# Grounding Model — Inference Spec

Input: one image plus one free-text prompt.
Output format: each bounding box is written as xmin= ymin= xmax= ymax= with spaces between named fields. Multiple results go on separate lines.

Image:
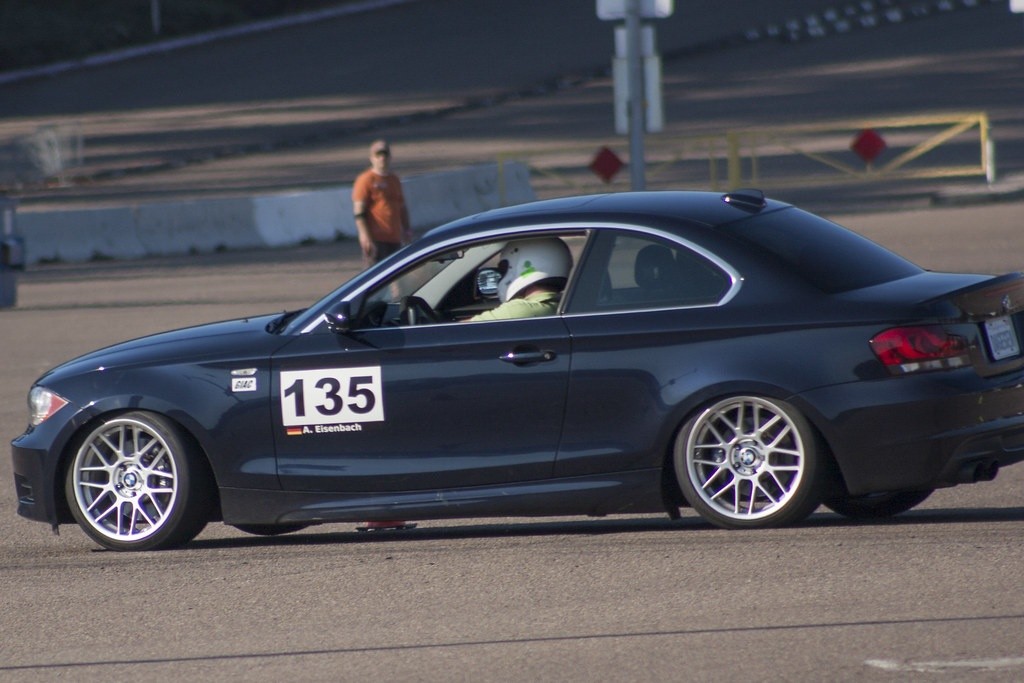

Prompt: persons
xmin=352 ymin=140 xmax=413 ymax=266
xmin=459 ymin=237 xmax=574 ymax=322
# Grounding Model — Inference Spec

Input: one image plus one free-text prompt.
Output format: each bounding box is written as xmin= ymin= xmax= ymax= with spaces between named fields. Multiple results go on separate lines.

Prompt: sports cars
xmin=9 ymin=186 xmax=1023 ymax=555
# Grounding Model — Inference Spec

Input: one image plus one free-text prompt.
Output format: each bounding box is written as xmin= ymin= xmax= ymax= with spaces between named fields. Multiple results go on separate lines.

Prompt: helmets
xmin=497 ymin=236 xmax=573 ymax=304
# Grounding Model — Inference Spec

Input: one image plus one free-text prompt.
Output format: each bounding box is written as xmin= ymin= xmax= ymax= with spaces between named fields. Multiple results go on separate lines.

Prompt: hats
xmin=371 ymin=140 xmax=390 ymax=155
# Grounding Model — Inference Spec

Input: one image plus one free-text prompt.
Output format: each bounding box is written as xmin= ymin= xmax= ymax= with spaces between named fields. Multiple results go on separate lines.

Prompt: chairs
xmin=611 ymin=245 xmax=680 ymax=307
